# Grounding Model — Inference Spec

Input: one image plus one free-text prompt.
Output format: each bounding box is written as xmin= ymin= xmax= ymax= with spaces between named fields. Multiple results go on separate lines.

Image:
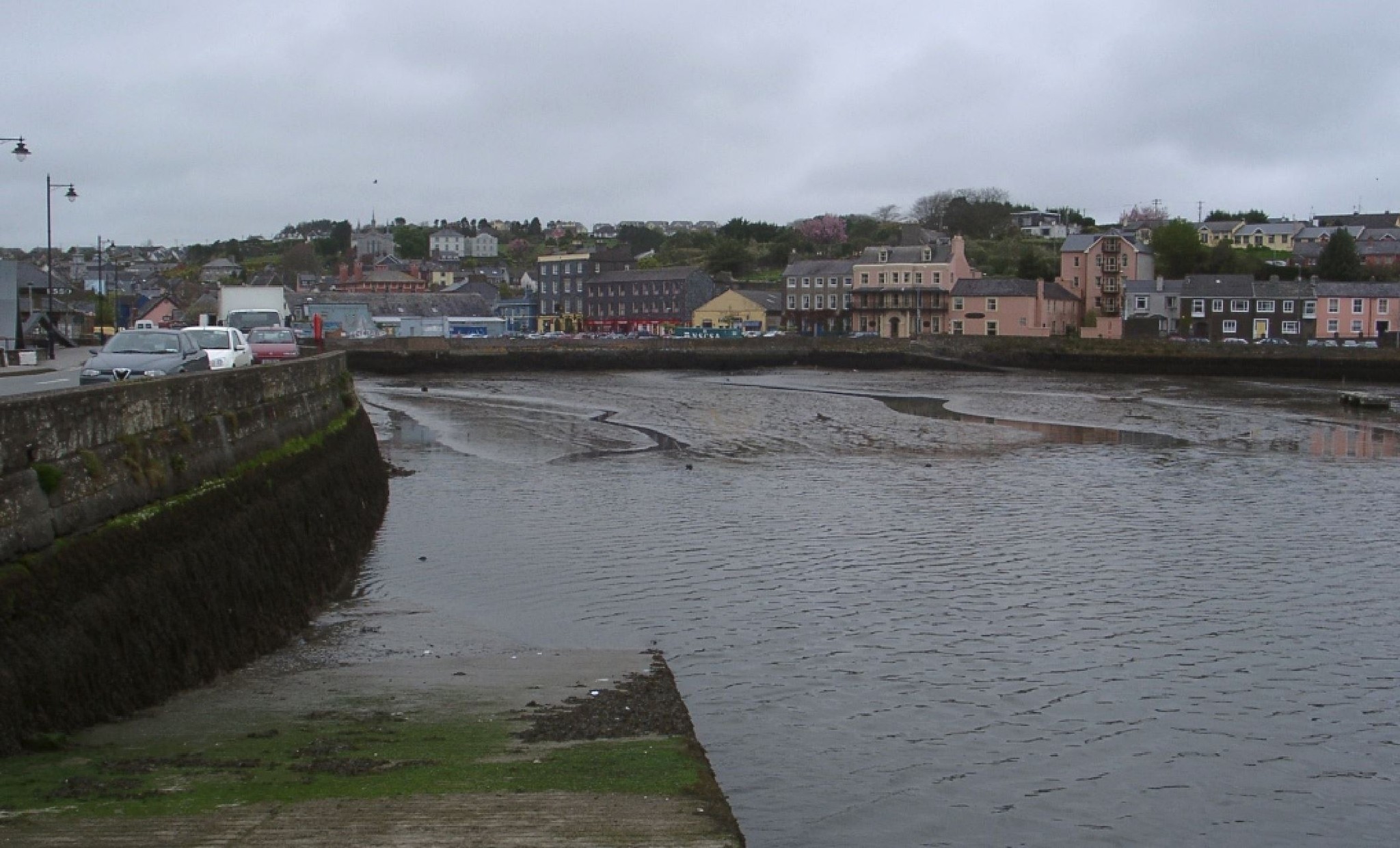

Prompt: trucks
xmin=215 ymin=284 xmax=288 ymax=345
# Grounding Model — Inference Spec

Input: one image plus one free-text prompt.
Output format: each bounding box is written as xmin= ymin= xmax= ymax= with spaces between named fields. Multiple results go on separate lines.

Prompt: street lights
xmin=97 ymin=233 xmax=117 ymax=351
xmin=46 ymin=173 xmax=80 ymax=359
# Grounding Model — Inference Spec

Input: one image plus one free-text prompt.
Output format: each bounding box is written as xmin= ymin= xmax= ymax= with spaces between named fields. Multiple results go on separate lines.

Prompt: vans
xmin=134 ymin=319 xmax=153 ymax=330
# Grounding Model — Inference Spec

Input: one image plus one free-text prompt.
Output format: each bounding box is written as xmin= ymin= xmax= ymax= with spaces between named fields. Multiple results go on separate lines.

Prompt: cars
xmin=839 ymin=331 xmax=878 ymax=340
xmin=520 ymin=330 xmax=663 ymax=340
xmin=1306 ymin=340 xmax=1318 ymax=346
xmin=1362 ymin=340 xmax=1379 ymax=349
xmin=242 ymin=326 xmax=300 ymax=367
xmin=176 ymin=325 xmax=254 ymax=373
xmin=78 ymin=328 xmax=211 ymax=386
xmin=1341 ymin=340 xmax=1359 ymax=348
xmin=1224 ymin=336 xmax=1291 ymax=345
xmin=461 ymin=333 xmax=486 ymax=339
xmin=743 ymin=329 xmax=786 ymax=338
xmin=1325 ymin=340 xmax=1340 ymax=347
